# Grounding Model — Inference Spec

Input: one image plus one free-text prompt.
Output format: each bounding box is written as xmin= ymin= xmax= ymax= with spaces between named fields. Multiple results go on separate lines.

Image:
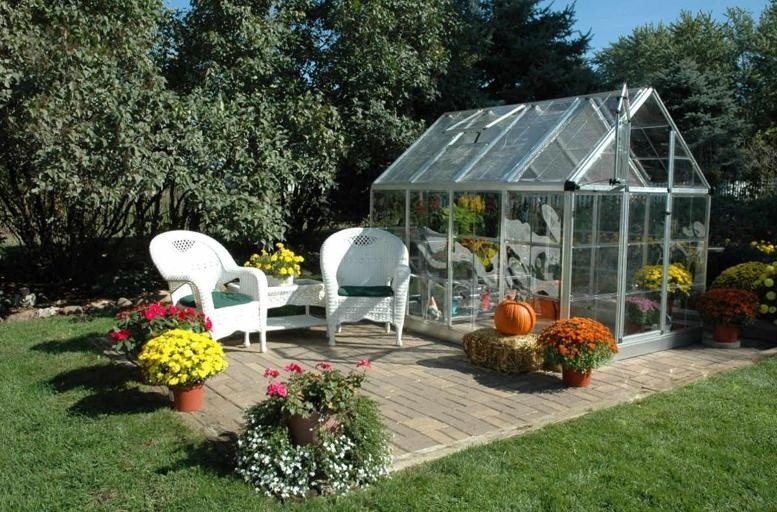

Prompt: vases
xmin=287 ymin=410 xmax=350 ymax=456
xmin=171 ymin=383 xmax=205 ymax=413
xmin=266 ymin=274 xmax=294 ymax=287
xmin=713 ymin=318 xmax=740 ymax=343
xmin=560 ymin=360 xmax=592 ymax=388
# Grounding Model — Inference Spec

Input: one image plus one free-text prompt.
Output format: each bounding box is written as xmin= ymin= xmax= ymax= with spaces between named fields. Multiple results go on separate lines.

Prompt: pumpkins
xmin=494 ymin=300 xmax=536 ymax=336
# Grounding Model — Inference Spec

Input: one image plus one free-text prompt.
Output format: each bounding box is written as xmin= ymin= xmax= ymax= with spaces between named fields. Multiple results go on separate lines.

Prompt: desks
xmin=228 ymin=279 xmax=328 ymax=349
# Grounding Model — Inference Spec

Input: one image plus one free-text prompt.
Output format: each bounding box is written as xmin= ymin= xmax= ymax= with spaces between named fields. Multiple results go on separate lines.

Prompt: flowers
xmin=536 ymin=316 xmax=620 ymax=376
xmin=697 ymin=288 xmax=761 ymax=326
xmin=625 ymin=261 xmax=694 ymax=327
xmin=259 ymin=354 xmax=371 ymax=417
xmin=107 ymin=301 xmax=231 ymax=391
xmin=243 ymin=240 xmax=305 ymax=279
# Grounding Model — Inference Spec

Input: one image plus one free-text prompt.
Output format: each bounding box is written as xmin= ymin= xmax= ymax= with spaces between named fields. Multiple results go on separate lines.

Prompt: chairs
xmin=147 ymin=228 xmax=269 ymax=354
xmin=319 ymin=225 xmax=413 ymax=348
